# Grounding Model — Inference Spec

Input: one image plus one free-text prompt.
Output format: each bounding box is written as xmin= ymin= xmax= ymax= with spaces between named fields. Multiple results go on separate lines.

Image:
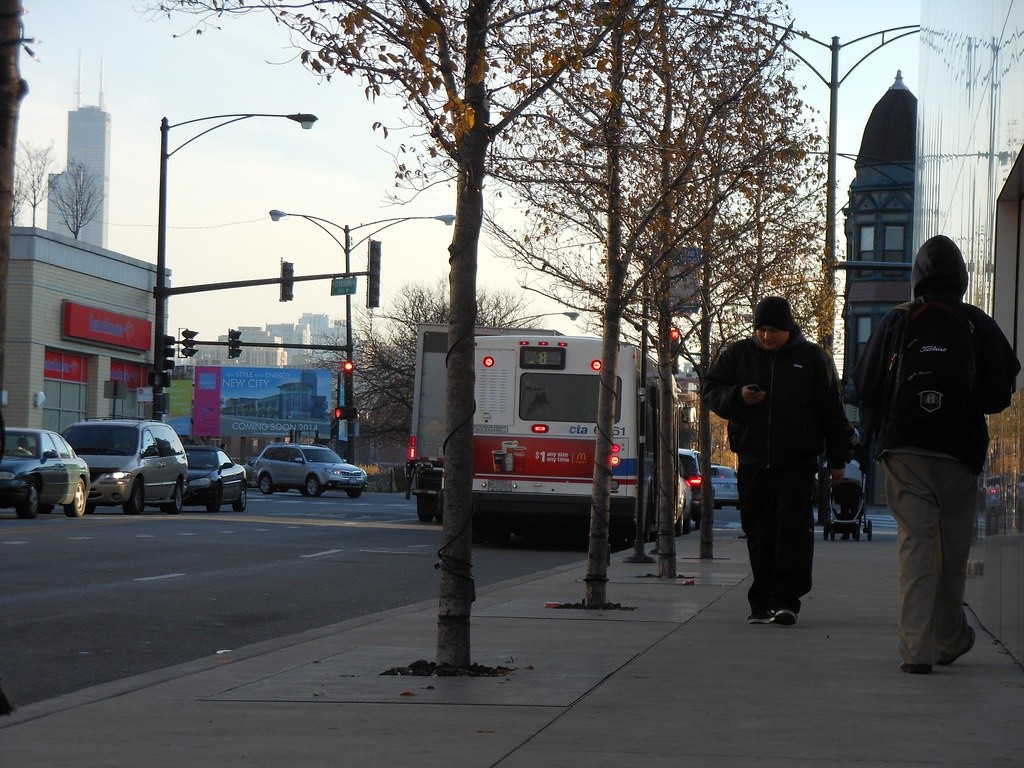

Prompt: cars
xmin=240 ymin=456 xmax=289 ymax=493
xmin=0 ymin=427 xmax=91 ymax=520
xmin=182 ymin=444 xmax=248 ymax=513
xmin=675 ymin=445 xmax=741 ymax=538
xmin=984 ymin=474 xmax=1024 ymax=508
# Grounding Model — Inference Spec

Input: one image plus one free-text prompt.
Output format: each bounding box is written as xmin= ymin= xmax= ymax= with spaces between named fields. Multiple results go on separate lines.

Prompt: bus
xmin=472 ymin=334 xmax=682 ymax=547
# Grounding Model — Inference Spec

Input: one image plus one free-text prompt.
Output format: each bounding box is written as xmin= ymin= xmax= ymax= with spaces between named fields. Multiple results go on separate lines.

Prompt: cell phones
xmin=748 ymin=384 xmax=760 ymax=393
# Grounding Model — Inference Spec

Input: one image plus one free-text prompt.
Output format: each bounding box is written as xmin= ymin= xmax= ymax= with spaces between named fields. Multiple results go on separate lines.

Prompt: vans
xmin=56 ymin=415 xmax=188 ymax=515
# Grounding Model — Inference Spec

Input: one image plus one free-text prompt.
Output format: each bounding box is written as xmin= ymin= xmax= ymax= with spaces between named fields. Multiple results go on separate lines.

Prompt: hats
xmin=754 ymin=297 xmax=792 ymax=329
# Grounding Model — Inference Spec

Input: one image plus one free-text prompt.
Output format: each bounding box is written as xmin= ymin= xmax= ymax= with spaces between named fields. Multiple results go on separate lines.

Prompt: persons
xmin=841 ymin=428 xmax=864 ymax=541
xmin=702 ymin=296 xmax=850 ymax=624
xmin=851 ymin=234 xmax=1022 ymax=675
xmin=18 ymin=437 xmax=35 ymax=454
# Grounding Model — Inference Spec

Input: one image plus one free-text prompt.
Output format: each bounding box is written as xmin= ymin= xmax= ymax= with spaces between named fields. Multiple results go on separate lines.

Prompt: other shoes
xmin=776 ymin=608 xmax=797 ymax=625
xmin=841 ymin=532 xmax=850 ymax=540
xmin=934 ymin=625 xmax=974 ymax=666
xmin=748 ymin=606 xmax=775 ymax=624
xmin=899 ymin=661 xmax=931 ymax=673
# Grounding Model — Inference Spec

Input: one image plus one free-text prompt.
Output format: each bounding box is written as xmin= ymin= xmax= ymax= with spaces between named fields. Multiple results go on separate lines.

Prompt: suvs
xmin=254 ymin=442 xmax=368 ymax=499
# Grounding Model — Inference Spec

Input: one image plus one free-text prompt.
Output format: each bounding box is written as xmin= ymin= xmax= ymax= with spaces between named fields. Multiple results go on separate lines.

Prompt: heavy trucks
xmin=406 ymin=323 xmax=566 ymax=523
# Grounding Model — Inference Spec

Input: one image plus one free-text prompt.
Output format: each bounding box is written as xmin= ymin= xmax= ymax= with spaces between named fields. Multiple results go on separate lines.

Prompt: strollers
xmin=823 ymin=440 xmax=874 ymax=542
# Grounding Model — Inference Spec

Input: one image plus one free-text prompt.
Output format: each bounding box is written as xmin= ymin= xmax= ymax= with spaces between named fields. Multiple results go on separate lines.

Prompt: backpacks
xmin=876 ymin=301 xmax=980 ymax=445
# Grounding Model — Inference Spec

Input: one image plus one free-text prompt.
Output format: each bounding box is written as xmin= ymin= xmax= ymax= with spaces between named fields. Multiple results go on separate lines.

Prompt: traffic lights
xmin=345 ymin=362 xmax=353 ymax=406
xmin=229 ymin=329 xmax=243 ymax=358
xmin=152 ymin=371 xmax=171 ymax=387
xmin=160 ymin=334 xmax=176 ymax=369
xmin=335 ymin=407 xmax=347 ymax=419
xmin=181 ymin=329 xmax=200 ymax=357
xmin=670 ymin=330 xmax=679 ymax=372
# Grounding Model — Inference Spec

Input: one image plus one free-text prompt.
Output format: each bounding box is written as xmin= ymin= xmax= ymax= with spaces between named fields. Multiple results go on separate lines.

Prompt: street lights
xmin=269 ymin=209 xmax=458 ymax=463
xmin=152 ymin=110 xmax=317 ymax=421
